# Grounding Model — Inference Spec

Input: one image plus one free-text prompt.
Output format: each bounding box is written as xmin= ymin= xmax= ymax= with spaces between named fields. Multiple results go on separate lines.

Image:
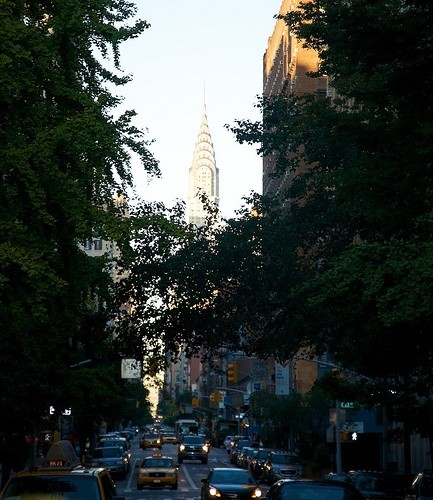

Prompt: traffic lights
xmin=340 ymin=430 xmax=347 ymax=442
xmin=227 ymin=362 xmax=238 ymax=386
xmin=348 ymin=431 xmax=359 ymax=443
xmin=210 ymin=391 xmax=224 ymax=405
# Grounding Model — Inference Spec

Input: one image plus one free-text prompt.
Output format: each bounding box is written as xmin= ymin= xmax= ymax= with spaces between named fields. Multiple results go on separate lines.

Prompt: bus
xmin=175 ymin=419 xmax=199 ymax=442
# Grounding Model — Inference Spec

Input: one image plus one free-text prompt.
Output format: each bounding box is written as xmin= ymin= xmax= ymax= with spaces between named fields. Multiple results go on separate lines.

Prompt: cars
xmin=97 ymin=419 xmax=178 ymax=454
xmin=200 ymin=468 xmax=267 ymax=500
xmin=224 ymin=435 xmax=274 ymax=476
xmin=198 ymin=433 xmax=207 ymax=439
xmin=343 ymin=469 xmax=390 ymax=500
xmin=405 ymin=470 xmax=433 ymax=500
xmin=85 ymin=445 xmax=129 ymax=479
xmin=263 ymin=478 xmax=367 ymax=500
xmin=135 ymin=453 xmax=180 ymax=489
xmin=0 ymin=440 xmax=127 ymax=500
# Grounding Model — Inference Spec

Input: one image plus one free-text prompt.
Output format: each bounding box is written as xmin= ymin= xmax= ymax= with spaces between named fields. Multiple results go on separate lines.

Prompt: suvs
xmin=178 ymin=435 xmax=210 ymax=464
xmin=262 ymin=451 xmax=306 ymax=484
